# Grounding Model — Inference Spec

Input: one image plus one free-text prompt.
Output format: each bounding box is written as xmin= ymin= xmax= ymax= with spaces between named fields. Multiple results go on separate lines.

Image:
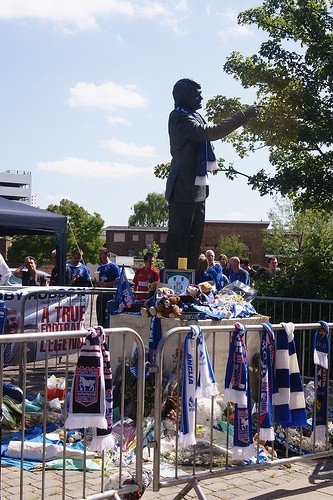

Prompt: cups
xmin=94 ymin=271 xmax=101 ymax=282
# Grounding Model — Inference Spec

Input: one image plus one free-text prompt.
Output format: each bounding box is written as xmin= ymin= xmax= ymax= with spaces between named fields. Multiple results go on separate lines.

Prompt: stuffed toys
xmin=141 ymin=282 xmax=213 ymax=318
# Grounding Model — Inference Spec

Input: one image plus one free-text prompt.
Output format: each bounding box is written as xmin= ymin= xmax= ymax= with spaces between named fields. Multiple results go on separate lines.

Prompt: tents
xmin=0 ymin=196 xmax=68 ymax=286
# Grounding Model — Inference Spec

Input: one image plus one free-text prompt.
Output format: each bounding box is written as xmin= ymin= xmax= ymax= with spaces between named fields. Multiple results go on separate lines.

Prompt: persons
xmin=163 ymin=78 xmax=266 ymax=269
xmin=1 ymin=309 xmax=35 ymax=367
xmin=0 ymin=249 xmax=286 ymax=327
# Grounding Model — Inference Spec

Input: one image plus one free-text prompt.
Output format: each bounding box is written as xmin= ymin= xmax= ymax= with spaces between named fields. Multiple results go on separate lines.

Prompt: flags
xmin=114 ymin=267 xmax=134 ymax=314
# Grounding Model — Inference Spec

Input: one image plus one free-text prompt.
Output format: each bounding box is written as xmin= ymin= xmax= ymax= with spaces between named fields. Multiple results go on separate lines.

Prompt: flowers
xmin=273 ymin=418 xmax=333 ymax=453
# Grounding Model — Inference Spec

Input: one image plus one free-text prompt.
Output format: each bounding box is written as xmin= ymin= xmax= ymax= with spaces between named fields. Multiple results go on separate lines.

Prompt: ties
xmin=4 ymin=343 xmax=12 ymax=363
